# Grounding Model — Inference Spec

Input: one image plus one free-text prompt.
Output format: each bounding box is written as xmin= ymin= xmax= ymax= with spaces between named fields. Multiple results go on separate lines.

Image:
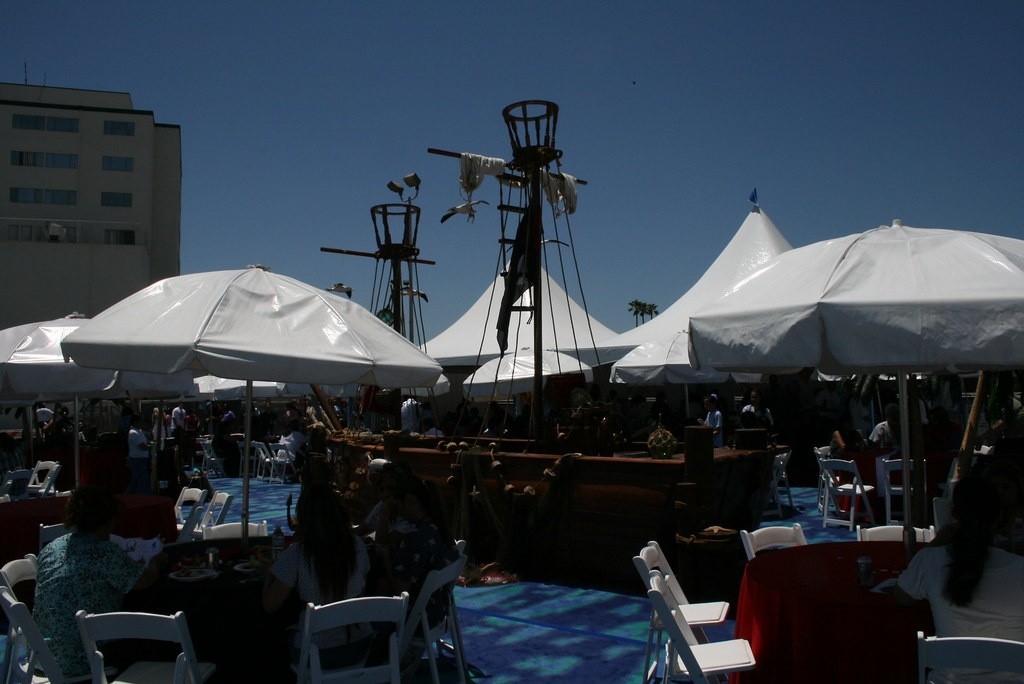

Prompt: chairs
xmin=0 ymin=460 xmax=63 ymax=501
xmin=819 ymin=458 xmax=875 ymax=531
xmin=237 ymin=441 xmax=298 ymax=484
xmin=646 ymin=569 xmax=756 ymax=684
xmin=292 ymin=538 xmax=469 ymax=684
xmin=740 ymin=523 xmax=807 ymax=562
xmin=856 ymin=525 xmax=936 ymax=543
xmin=202 ymin=441 xmax=228 ymax=478
xmin=762 ymin=450 xmax=794 ymax=517
xmin=0 ymin=522 xmax=216 ymax=684
xmin=917 ymin=630 xmax=1024 ymax=684
xmin=175 ymin=487 xmax=268 ymax=540
xmin=814 ymin=446 xmax=841 ymax=516
xmin=632 ymin=540 xmax=729 ymax=684
xmin=882 ymin=458 xmax=927 ymax=528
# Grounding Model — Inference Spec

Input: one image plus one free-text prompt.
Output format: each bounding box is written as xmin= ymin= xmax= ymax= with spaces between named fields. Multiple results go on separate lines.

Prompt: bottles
xmin=205 ymin=547 xmax=219 ymax=570
xmin=271 ymin=525 xmax=285 ymax=563
xmin=857 ymin=556 xmax=873 ymax=590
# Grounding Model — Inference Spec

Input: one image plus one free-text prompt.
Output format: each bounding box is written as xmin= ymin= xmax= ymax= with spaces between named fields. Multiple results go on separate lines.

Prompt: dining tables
xmin=103 ymin=538 xmax=394 ymax=684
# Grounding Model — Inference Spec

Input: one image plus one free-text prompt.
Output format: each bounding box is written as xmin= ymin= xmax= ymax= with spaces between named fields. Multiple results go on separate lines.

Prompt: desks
xmin=832 ymin=446 xmax=995 ymax=525
xmin=73 ymin=447 xmax=175 ymax=492
xmin=0 ymin=496 xmax=180 ymax=620
xmin=729 ymin=540 xmax=935 ymax=684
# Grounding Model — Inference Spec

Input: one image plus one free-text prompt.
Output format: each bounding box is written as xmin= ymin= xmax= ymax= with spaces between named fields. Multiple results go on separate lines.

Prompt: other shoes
xmin=399 ymin=656 xmax=420 ymax=684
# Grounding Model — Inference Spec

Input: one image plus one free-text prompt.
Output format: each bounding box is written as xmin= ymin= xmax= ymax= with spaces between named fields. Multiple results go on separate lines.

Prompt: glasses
xmin=889 ymin=412 xmax=899 ymax=416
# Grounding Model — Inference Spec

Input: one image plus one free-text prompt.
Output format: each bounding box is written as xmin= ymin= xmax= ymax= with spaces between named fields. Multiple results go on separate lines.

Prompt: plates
xmin=177 ymin=559 xmax=223 ymax=568
xmin=168 ymin=568 xmax=211 ymax=582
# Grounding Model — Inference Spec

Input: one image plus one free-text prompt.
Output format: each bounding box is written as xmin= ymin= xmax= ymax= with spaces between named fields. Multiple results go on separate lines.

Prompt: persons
xmin=741 ymin=388 xmax=774 ymax=426
xmin=399 ymin=395 xmax=422 ymax=433
xmin=152 ymin=406 xmax=166 ymax=440
xmin=830 ymin=416 xmax=867 ymax=476
xmin=922 ymin=407 xmax=963 ymax=449
xmin=171 ymin=402 xmax=186 ymax=434
xmin=354 ymin=464 xmax=460 ymax=684
xmin=0 ymin=432 xmax=29 ymax=495
xmin=212 ymin=425 xmax=240 ymax=457
xmin=624 ymin=393 xmax=650 ymax=438
xmin=269 ymin=482 xmax=375 ymax=670
xmin=893 ymin=477 xmax=1024 ymax=641
xmin=259 ymin=395 xmax=279 ymax=434
xmin=696 ymin=394 xmax=723 ymax=447
xmin=125 ymin=415 xmax=152 ymax=495
xmin=450 ymin=398 xmax=531 ymax=438
xmin=182 ymin=409 xmax=203 ymax=436
xmin=650 ymin=391 xmax=672 ymax=428
xmin=32 ymin=481 xmax=160 ymax=677
xmin=868 ymin=402 xmax=901 ymax=444
xmin=985 ymin=467 xmax=1018 ymax=502
xmin=284 ymin=399 xmax=300 ymax=423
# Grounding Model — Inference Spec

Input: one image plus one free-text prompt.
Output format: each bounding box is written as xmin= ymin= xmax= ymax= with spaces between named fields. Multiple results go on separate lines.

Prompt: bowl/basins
xmin=233 ymin=563 xmax=253 ymax=585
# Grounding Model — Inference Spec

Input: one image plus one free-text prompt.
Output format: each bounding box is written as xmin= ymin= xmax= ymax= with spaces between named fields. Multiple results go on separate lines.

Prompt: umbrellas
xmin=424 ymin=259 xmax=634 ymax=396
xmin=0 ymin=311 xmax=451 ymax=492
xmin=610 ymin=188 xmax=1024 ymax=565
xmin=60 ymin=261 xmax=443 ymax=539
